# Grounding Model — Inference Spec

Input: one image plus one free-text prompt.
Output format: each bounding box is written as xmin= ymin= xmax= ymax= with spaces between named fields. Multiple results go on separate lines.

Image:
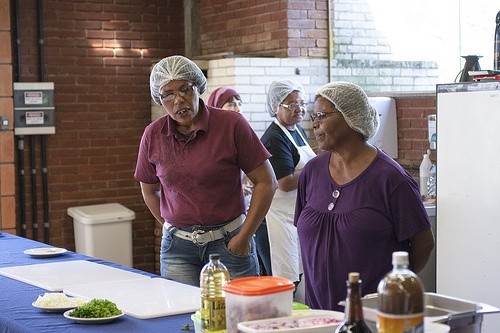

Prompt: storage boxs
xmin=223 ymin=275 xmax=295 ymax=333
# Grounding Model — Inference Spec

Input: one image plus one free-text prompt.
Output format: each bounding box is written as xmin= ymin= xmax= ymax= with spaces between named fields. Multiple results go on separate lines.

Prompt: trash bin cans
xmin=66 ymin=202 xmax=136 ymax=267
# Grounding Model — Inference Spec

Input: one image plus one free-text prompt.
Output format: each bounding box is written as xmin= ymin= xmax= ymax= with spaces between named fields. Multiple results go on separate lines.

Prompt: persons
xmin=253 ymin=217 xmax=272 ymax=276
xmin=293 ymin=81 xmax=434 ymax=313
xmin=260 ymin=79 xmax=317 ymax=298
xmin=134 ymin=56 xmax=278 ymax=287
xmin=207 ymin=87 xmax=254 ymax=214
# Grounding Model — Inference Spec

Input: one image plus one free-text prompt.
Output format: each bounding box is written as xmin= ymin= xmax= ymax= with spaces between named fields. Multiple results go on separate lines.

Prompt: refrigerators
xmin=436 ymin=79 xmax=500 ymax=333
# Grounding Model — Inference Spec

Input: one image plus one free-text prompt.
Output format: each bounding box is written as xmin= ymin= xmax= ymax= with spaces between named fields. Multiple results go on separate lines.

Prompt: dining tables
xmin=0 ymin=232 xmax=201 ymax=333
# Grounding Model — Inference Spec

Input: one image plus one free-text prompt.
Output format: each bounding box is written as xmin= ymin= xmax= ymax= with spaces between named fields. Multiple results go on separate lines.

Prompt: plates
xmin=31 ymin=297 xmax=88 ymax=312
xmin=22 ymin=248 xmax=68 ymax=257
xmin=63 ymin=309 xmax=125 ymax=323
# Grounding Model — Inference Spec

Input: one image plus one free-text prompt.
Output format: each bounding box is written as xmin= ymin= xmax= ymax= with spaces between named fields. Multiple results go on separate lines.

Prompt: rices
xmin=35 ymin=292 xmax=71 ymax=308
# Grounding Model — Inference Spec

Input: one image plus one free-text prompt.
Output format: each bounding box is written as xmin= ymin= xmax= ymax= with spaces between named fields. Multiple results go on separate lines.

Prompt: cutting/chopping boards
xmin=0 ymin=259 xmax=200 ymax=320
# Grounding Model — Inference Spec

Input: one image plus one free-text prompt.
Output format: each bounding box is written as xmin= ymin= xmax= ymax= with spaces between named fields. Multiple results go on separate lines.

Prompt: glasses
xmin=280 ymin=102 xmax=309 ymax=110
xmin=310 ymin=110 xmax=339 ymax=123
xmin=159 ymin=83 xmax=196 ymax=102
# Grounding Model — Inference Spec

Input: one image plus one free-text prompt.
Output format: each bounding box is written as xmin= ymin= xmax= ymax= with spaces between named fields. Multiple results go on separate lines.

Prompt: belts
xmin=164 ymin=213 xmax=247 ymax=247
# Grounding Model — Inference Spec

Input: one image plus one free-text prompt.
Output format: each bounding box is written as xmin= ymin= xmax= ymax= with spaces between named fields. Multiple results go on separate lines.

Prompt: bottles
xmin=334 ymin=272 xmax=374 ymax=333
xmin=200 ymin=254 xmax=231 ymax=333
xmin=419 ymin=149 xmax=436 ymax=199
xmin=376 ymin=251 xmax=424 ymax=333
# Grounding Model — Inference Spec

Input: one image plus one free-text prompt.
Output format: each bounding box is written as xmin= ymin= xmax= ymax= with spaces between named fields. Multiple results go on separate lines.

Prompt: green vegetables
xmin=68 ymin=298 xmax=123 ymax=318
